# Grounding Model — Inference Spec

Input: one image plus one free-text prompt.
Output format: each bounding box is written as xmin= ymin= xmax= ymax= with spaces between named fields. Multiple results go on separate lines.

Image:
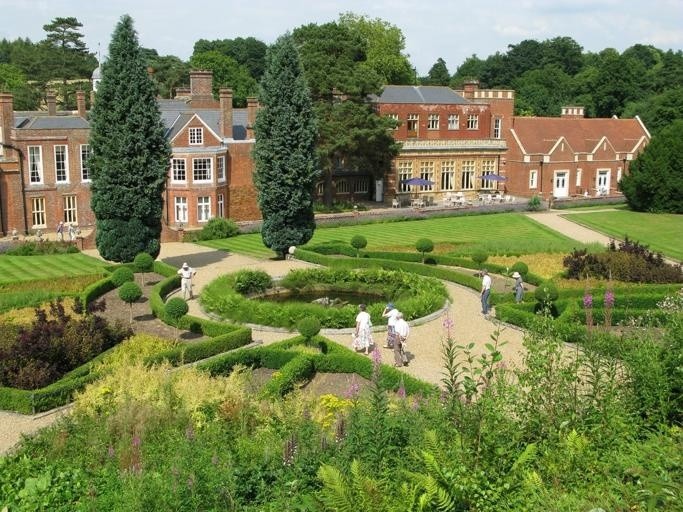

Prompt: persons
xmin=68 ymin=224 xmax=81 ymax=240
xmin=177 ymin=262 xmax=197 ymax=299
xmin=352 ymin=303 xmax=373 ymax=355
xmin=382 ymin=303 xmax=410 ymax=367
xmin=512 ymin=272 xmax=524 ymax=303
xmin=57 ymin=221 xmax=63 ymax=240
xmin=480 ymin=269 xmax=491 ymax=314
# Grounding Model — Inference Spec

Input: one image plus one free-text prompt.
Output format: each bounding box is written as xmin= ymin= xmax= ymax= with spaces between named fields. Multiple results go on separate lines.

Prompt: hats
xmin=512 ymin=272 xmax=521 ymax=278
xmin=183 ymin=263 xmax=189 ymax=269
xmin=386 ymin=303 xmax=394 ymax=310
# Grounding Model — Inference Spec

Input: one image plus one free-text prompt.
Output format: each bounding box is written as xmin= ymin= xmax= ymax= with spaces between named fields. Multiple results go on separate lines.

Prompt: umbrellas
xmin=476 ymin=174 xmax=511 ymax=181
xmin=399 ymin=177 xmax=436 ymax=198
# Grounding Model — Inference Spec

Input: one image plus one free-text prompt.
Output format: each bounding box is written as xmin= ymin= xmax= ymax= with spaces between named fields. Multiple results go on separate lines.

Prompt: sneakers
xmin=383 ymin=343 xmax=391 ymax=348
